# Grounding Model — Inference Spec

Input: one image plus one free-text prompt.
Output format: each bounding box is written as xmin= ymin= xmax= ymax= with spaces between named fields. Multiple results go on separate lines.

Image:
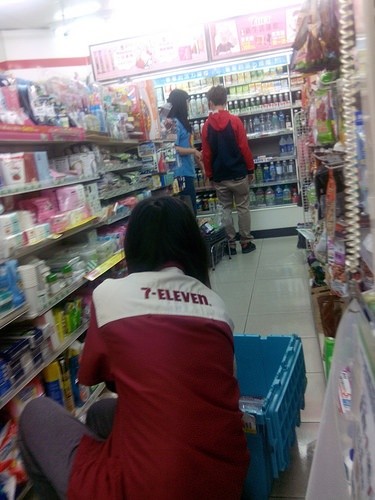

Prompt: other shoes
xmin=224 ymin=245 xmax=237 ymax=255
xmin=242 ymin=242 xmax=256 ymax=253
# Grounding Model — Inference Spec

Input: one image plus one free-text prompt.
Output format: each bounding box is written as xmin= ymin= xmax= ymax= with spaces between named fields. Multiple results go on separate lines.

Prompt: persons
xmin=202 ymin=85 xmax=257 ymax=256
xmin=17 ymin=195 xmax=251 ymax=500
xmin=162 ymin=88 xmax=204 ymax=226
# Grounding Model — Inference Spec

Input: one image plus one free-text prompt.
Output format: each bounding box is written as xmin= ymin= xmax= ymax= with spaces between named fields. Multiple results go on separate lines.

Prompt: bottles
xmin=159 ymin=89 xmax=301 ymax=213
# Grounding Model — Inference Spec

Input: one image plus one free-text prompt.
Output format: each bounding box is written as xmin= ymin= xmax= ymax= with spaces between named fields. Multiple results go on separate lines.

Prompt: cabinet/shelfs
xmin=150 ymin=52 xmax=332 ymax=236
xmin=0 ymin=75 xmax=181 ymax=500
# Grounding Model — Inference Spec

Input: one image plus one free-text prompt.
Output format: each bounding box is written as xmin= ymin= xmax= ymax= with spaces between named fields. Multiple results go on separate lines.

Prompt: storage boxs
xmin=228 ymin=333 xmax=309 ymax=500
xmin=196 ymin=228 xmax=228 ymax=266
